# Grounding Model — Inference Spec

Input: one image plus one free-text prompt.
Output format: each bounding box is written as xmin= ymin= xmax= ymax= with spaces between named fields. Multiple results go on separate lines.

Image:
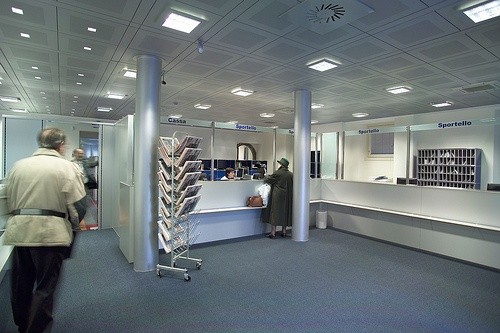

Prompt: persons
xmin=264 ymin=158 xmax=293 ymax=238
xmin=0 ymin=128 xmax=86 ymax=333
xmin=70 ymin=148 xmax=98 ymax=183
xmin=221 ymin=168 xmax=234 ymax=180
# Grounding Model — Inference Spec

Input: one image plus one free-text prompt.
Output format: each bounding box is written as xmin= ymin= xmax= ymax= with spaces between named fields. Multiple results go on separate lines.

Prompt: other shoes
xmin=266 ymin=233 xmax=275 ymax=238
xmin=280 ymin=232 xmax=286 ymax=236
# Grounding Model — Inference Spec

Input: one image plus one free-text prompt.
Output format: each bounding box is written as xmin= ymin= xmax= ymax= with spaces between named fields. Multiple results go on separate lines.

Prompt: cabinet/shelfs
xmin=415 ymin=148 xmax=481 ymax=189
xmin=199 ymin=159 xmax=267 ymax=181
xmin=310 ymin=150 xmax=320 ymax=178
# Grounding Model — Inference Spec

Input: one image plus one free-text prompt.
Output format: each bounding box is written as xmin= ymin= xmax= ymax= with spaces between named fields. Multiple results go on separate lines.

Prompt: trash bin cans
xmin=316 ymin=209 xmax=327 ymax=229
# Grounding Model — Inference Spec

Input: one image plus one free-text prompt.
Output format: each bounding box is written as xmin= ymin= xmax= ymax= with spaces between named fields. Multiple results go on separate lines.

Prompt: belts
xmin=11 ymin=207 xmax=66 ymax=217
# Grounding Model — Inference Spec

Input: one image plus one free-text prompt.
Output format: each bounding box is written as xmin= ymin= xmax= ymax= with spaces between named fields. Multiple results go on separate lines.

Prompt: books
xmin=157 ymin=136 xmax=203 ymax=254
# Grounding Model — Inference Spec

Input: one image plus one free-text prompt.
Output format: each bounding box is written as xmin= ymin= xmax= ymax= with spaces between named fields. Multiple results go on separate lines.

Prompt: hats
xmin=277 ymin=157 xmax=289 ymax=168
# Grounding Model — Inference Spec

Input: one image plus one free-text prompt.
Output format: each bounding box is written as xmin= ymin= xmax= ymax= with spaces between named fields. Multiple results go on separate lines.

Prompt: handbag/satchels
xmin=246 ymin=195 xmax=263 ymax=206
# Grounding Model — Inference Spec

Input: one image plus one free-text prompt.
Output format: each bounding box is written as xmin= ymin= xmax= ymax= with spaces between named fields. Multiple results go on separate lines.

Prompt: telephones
xmin=375 ymin=176 xmax=388 ymax=182
